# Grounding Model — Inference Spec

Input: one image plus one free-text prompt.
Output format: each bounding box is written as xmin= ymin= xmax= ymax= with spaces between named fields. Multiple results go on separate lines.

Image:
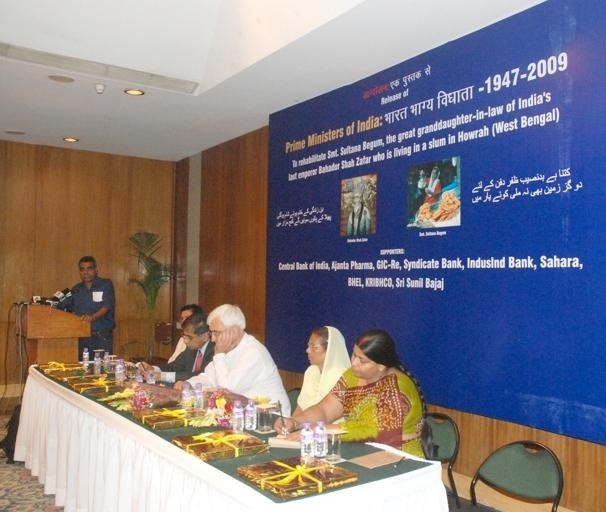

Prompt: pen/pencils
xmin=140 ymin=359 xmax=146 ymax=371
xmin=278 ymin=400 xmax=288 ymax=436
xmin=394 ymin=456 xmax=405 ymax=468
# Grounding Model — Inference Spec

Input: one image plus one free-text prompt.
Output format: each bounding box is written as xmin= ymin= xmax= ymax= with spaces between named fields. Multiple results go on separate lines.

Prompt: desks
xmin=12 ymin=361 xmax=448 ymax=512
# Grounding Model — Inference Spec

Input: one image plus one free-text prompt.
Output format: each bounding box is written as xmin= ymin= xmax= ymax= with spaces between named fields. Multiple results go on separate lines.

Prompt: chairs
xmin=283 ymin=388 xmax=302 ymax=414
xmin=470 ymin=439 xmax=566 ymax=511
xmin=421 ymin=412 xmax=462 ymax=512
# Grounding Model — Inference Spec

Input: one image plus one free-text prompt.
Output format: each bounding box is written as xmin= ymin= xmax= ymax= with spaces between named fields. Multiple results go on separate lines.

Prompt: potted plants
xmin=126 ymin=230 xmax=170 ymax=366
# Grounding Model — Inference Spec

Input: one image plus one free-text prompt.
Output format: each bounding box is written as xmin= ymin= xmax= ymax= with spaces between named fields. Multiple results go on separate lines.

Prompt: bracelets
xmin=290 ymin=419 xmax=298 ymax=431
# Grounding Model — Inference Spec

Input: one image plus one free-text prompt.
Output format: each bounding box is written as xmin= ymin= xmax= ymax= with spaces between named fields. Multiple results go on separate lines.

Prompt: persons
xmin=56 ymin=256 xmax=116 ymax=361
xmin=134 ymin=304 xmax=202 ymax=369
xmin=290 ymin=326 xmax=352 ymax=419
xmin=418 ymin=167 xmax=442 ymax=205
xmin=173 ymin=303 xmax=292 ymax=418
xmin=274 ymin=328 xmax=433 ymax=461
xmin=346 ymin=184 xmax=371 ymax=236
xmin=136 ymin=312 xmax=215 ymax=388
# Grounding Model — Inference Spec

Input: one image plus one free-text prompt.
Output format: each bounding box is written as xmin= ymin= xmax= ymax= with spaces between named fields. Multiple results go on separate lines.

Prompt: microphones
xmin=30 ymin=287 xmax=80 ymax=313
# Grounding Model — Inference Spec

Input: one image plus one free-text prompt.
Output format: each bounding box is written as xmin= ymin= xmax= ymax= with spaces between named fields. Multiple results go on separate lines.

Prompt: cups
xmin=326 ymin=432 xmax=342 ymax=460
xmin=257 ymin=407 xmax=272 ymax=431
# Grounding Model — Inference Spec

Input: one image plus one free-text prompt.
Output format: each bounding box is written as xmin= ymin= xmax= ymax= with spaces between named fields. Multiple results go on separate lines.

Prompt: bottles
xmin=314 ymin=420 xmax=328 ymax=458
xmin=147 ymin=366 xmax=156 ymax=385
xmin=181 ymin=384 xmax=194 ymax=409
xmin=300 ymin=422 xmax=315 ymax=459
xmin=136 ymin=368 xmax=144 ymax=384
xmin=194 ymin=382 xmax=204 ymax=412
xmin=94 ymin=353 xmax=101 ymax=376
xmin=115 ymin=358 xmax=126 ymax=383
xmin=232 ymin=400 xmax=244 ymax=433
xmin=82 ymin=348 xmax=90 ymax=369
xmin=103 ymin=352 xmax=111 ymax=373
xmin=244 ymin=399 xmax=257 ymax=431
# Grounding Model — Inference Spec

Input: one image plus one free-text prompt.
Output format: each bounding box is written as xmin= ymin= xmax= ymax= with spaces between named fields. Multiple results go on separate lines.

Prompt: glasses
xmin=180 ymin=334 xmax=198 ymax=340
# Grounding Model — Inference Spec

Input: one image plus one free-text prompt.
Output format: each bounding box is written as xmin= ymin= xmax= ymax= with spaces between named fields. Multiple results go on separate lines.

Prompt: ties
xmin=195 ymin=350 xmax=201 ymax=374
